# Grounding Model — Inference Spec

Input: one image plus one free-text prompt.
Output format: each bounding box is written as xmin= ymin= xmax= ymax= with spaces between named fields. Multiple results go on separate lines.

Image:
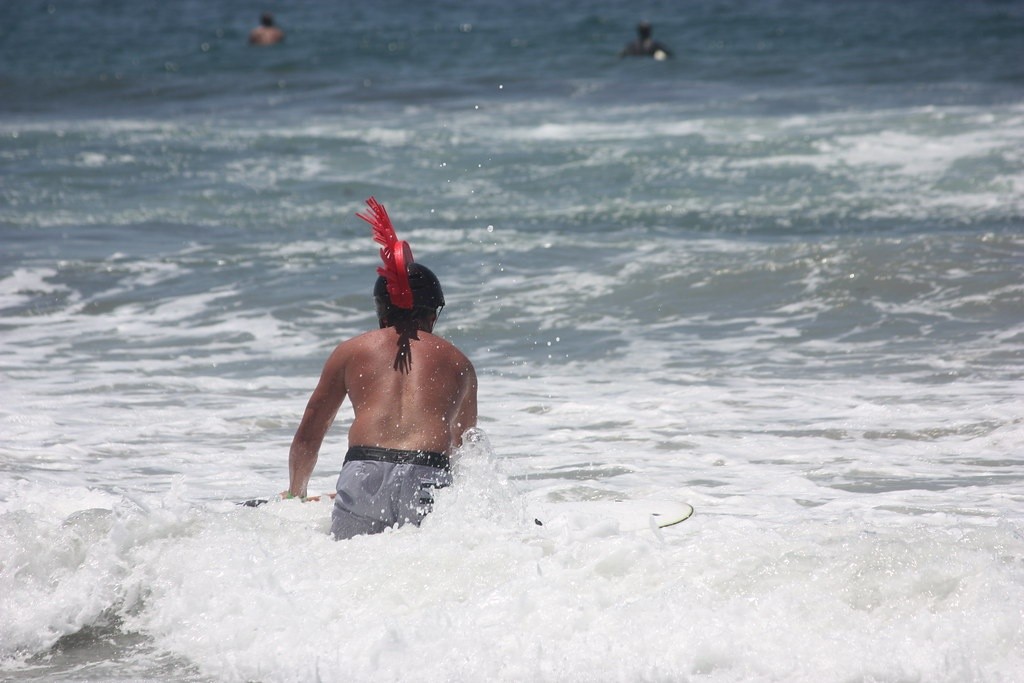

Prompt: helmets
xmin=356 ymin=196 xmax=445 ymax=318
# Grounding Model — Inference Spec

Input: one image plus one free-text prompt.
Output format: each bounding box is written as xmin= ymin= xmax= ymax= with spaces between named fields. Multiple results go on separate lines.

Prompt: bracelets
xmin=287 ymin=489 xmax=307 ymax=502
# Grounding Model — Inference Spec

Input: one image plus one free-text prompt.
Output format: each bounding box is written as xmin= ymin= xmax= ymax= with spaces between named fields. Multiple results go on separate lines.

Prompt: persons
xmin=618 ymin=20 xmax=672 ymax=59
xmin=281 ymin=261 xmax=477 ymax=541
xmin=249 ymin=16 xmax=284 ymax=45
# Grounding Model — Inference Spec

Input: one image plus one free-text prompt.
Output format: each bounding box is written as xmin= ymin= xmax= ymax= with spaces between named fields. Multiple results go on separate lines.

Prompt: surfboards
xmin=235 ymin=497 xmax=693 ymax=529
